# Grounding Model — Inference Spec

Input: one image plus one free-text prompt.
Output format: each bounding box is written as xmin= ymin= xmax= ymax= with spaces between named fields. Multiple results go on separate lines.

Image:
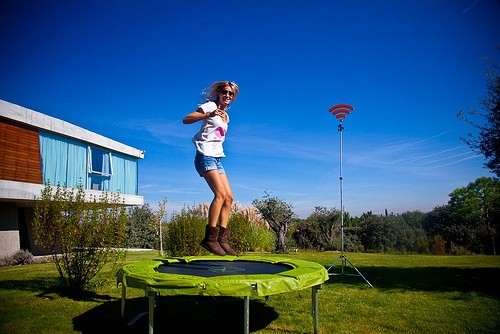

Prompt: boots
xmin=200 ymin=224 xmax=226 ymax=256
xmin=217 ymin=225 xmax=237 ymax=255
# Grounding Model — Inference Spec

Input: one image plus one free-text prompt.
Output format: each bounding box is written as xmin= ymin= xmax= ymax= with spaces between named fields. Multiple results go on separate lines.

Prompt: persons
xmin=182 ymin=82 xmax=240 ymax=255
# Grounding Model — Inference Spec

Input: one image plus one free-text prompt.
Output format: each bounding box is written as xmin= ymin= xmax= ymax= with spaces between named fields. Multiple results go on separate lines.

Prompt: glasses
xmin=219 ymin=90 xmax=234 ymax=96
